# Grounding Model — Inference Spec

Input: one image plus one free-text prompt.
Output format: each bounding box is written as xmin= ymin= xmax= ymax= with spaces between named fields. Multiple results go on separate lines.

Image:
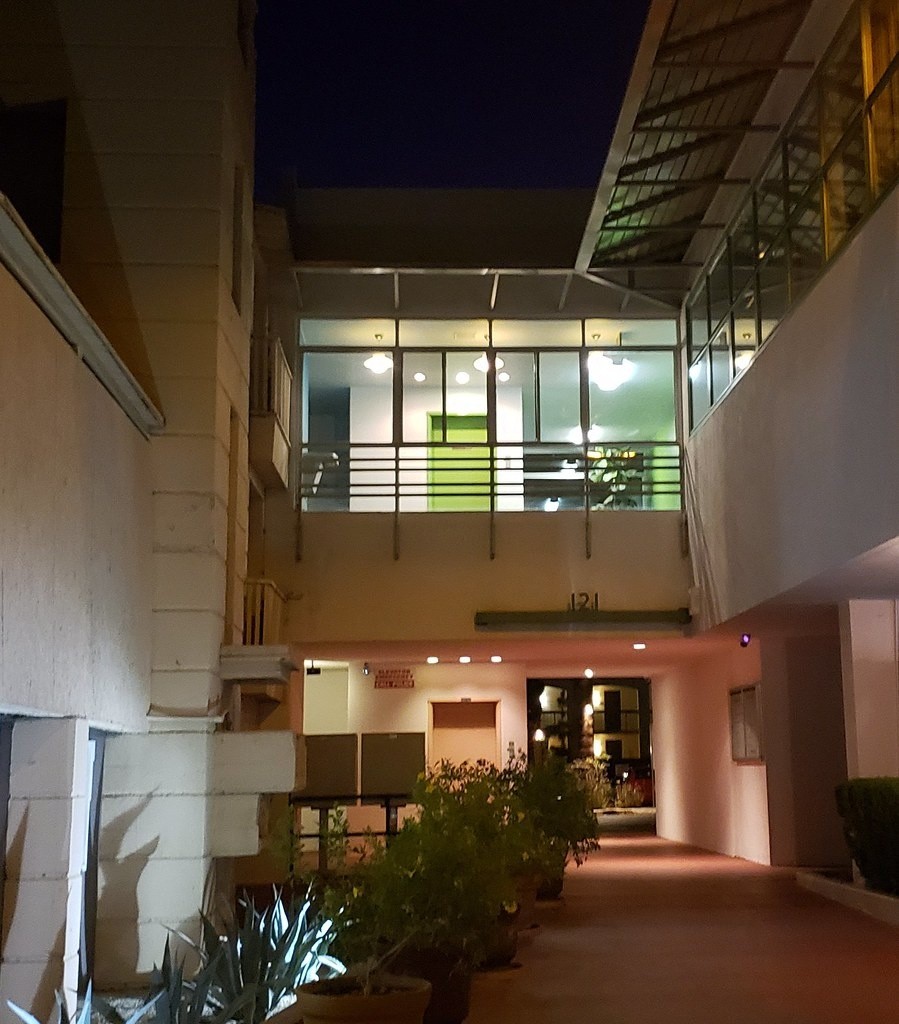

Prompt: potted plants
xmin=834 ymin=776 xmax=899 ymax=898
xmin=286 ymin=740 xmax=603 ymax=1024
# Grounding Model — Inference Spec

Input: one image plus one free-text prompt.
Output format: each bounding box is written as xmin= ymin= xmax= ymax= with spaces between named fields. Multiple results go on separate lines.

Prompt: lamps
xmin=306 ymin=660 xmax=321 ymax=674
xmin=362 ymin=663 xmax=369 ymax=675
xmin=677 ymin=607 xmax=693 ymax=624
xmin=740 ymin=632 xmax=751 ymax=647
